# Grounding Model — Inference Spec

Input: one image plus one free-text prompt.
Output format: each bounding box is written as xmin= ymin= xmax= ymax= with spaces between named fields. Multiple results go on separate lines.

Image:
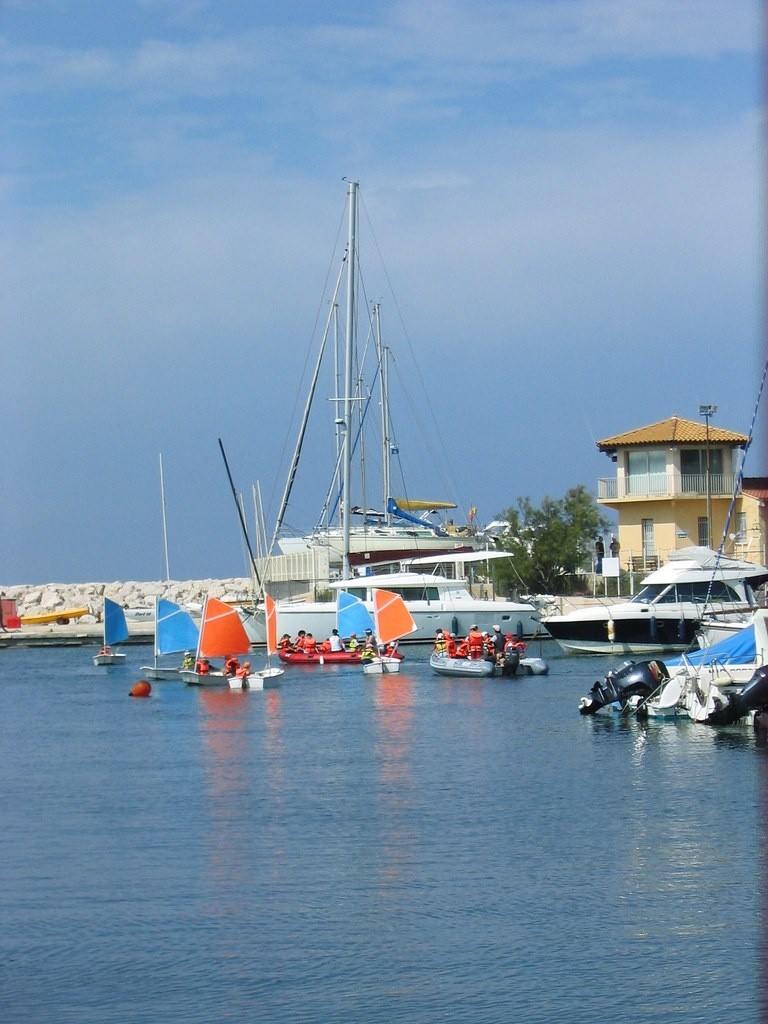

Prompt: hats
xmin=471 ymin=625 xmax=479 ymax=630
xmin=390 ymin=642 xmax=395 ymax=647
xmin=482 ymin=632 xmax=487 ymax=637
xmin=493 ymin=625 xmax=500 ymax=631
xmin=449 ymin=633 xmax=457 ymax=638
xmin=506 ymin=634 xmax=512 ymax=638
xmin=283 ymin=634 xmax=291 ymax=638
xmin=465 ymin=637 xmax=469 ymax=642
xmin=366 ymin=644 xmax=374 ymax=649
xmin=438 ymin=633 xmax=444 ymax=639
xmin=509 ymin=637 xmax=517 ymax=640
xmin=244 ymin=662 xmax=250 ymax=668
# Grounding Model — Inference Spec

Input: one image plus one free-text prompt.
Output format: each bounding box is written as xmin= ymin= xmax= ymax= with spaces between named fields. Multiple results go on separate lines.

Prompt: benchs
xmin=624 ymin=556 xmax=657 ymax=573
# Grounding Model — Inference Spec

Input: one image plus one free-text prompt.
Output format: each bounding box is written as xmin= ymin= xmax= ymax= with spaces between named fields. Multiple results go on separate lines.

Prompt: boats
xmin=519 ymin=657 xmax=549 ymax=676
xmin=19 ymin=607 xmax=89 ymax=626
xmin=429 ymin=646 xmax=495 ymax=678
xmin=181 ymin=592 xmax=252 ymax=687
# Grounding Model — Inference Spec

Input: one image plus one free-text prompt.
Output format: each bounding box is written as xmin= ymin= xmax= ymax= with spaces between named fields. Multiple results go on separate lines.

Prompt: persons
xmin=197 ymin=658 xmax=210 ymax=675
xmin=595 ymin=535 xmax=605 ymax=575
xmin=223 ymin=655 xmax=256 ymax=679
xmin=99 ymin=646 xmax=110 ymax=655
xmin=609 ymin=536 xmax=621 ymax=558
xmin=429 ymin=622 xmax=530 ymax=681
xmin=277 ymin=628 xmax=406 ymax=667
xmin=182 ymin=652 xmax=195 ymax=671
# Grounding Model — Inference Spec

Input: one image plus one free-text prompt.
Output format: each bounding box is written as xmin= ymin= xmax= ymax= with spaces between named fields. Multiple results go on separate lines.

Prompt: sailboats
xmin=362 ymin=587 xmax=418 ymax=674
xmin=277 ymin=588 xmax=405 ymax=663
xmin=139 ymin=594 xmax=200 ymax=680
xmin=239 ymin=175 xmax=544 ymax=645
xmin=538 ymin=541 xmax=768 ymax=656
xmin=92 ymin=595 xmax=129 ymax=666
xmin=580 ymin=358 xmax=768 ymax=725
xmin=228 ymin=590 xmax=286 ymax=689
